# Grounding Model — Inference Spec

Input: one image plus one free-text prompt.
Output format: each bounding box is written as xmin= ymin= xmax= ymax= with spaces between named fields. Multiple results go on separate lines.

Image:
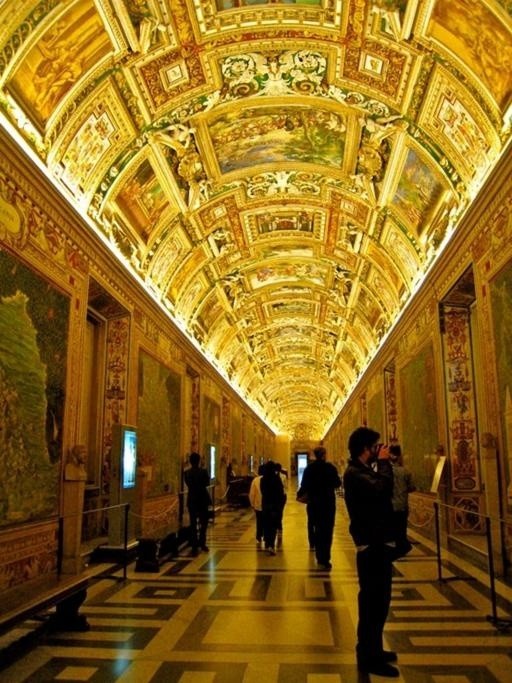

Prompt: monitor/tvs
xmin=210 ymin=446 xmax=216 ymax=479
xmin=122 ymin=430 xmax=137 ymax=489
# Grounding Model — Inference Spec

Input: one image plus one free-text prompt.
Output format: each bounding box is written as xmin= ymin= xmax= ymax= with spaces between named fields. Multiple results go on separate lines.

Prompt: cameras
xmin=372 ymin=443 xmax=402 ymax=463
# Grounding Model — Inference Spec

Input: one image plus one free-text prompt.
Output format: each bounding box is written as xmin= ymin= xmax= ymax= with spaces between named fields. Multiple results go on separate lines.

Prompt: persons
xmin=248 ymin=460 xmax=288 ymax=556
xmin=184 ymin=452 xmax=212 ymax=557
xmin=343 ymin=427 xmax=399 ymax=678
xmin=306 ymin=459 xmax=316 ymax=551
xmin=297 ymin=447 xmax=341 ymax=568
xmin=388 ymin=444 xmax=416 ymax=548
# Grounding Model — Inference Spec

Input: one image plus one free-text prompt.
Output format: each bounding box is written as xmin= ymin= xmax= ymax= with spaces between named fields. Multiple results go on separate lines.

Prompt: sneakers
xmin=358 ymin=650 xmax=399 ymax=677
xmin=318 ymin=560 xmax=331 ymax=568
